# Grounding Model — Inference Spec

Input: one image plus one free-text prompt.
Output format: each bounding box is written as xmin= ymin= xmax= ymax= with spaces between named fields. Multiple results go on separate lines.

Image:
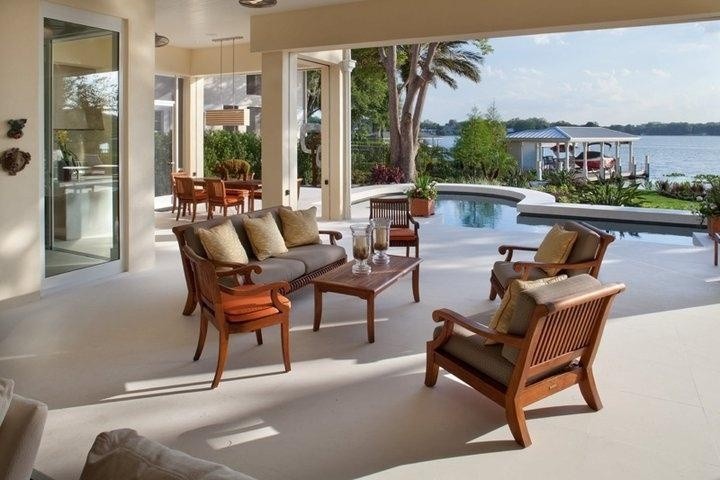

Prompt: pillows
xmin=533 ymin=226 xmax=577 ymax=277
xmin=0 ymin=375 xmax=16 ymax=426
xmin=241 ymin=212 xmax=288 ymax=260
xmin=80 ymin=428 xmax=254 ymax=480
xmin=278 ymin=206 xmax=320 ymax=247
xmin=485 ymin=274 xmax=568 ymax=348
xmin=197 ymin=220 xmax=250 ymax=266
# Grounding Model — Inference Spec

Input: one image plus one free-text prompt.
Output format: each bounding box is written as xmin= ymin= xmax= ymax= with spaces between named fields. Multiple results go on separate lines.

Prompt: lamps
xmin=155 ymin=33 xmax=169 ymax=47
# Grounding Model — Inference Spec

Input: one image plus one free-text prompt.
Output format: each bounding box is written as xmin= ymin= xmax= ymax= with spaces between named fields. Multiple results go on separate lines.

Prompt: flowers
xmin=54 ymin=130 xmax=75 ymax=162
xmin=696 ymin=175 xmax=720 ymax=230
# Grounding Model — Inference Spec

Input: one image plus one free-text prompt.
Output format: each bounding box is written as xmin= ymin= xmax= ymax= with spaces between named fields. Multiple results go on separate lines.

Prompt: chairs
xmin=425 ymin=273 xmax=626 ymax=448
xmin=370 ymin=198 xmax=420 ymax=259
xmin=489 ymin=223 xmax=614 ymax=300
xmin=543 ymin=156 xmax=566 ymax=169
xmin=175 ymin=177 xmax=208 ymax=222
xmin=248 ymin=179 xmax=262 ymax=212
xmin=171 ymin=172 xmax=193 ymax=215
xmin=182 ymin=245 xmax=291 ymax=390
xmin=204 ymin=178 xmax=244 ymax=220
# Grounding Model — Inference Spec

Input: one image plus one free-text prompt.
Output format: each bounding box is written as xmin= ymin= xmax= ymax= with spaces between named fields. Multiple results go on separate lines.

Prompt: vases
xmin=57 ymin=162 xmax=72 ymax=181
xmin=707 ymin=217 xmax=720 ymax=236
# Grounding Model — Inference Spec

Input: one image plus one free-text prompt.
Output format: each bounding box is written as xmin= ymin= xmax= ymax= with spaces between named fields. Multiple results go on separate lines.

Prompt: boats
xmin=575 ymin=151 xmax=616 ymax=171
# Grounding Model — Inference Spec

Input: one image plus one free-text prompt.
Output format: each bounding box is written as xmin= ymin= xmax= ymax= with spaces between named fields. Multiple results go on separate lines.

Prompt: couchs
xmin=171 ymin=206 xmax=347 ymax=332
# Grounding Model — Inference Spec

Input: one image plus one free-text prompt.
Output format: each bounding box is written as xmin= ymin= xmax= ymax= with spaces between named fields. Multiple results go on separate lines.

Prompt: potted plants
xmin=406 ymin=172 xmax=438 ymax=216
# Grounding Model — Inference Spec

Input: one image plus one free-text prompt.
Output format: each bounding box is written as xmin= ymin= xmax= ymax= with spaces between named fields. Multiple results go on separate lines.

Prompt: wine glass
xmin=370 ymin=217 xmax=393 ymax=266
xmin=350 ymin=223 xmax=373 ymax=275
